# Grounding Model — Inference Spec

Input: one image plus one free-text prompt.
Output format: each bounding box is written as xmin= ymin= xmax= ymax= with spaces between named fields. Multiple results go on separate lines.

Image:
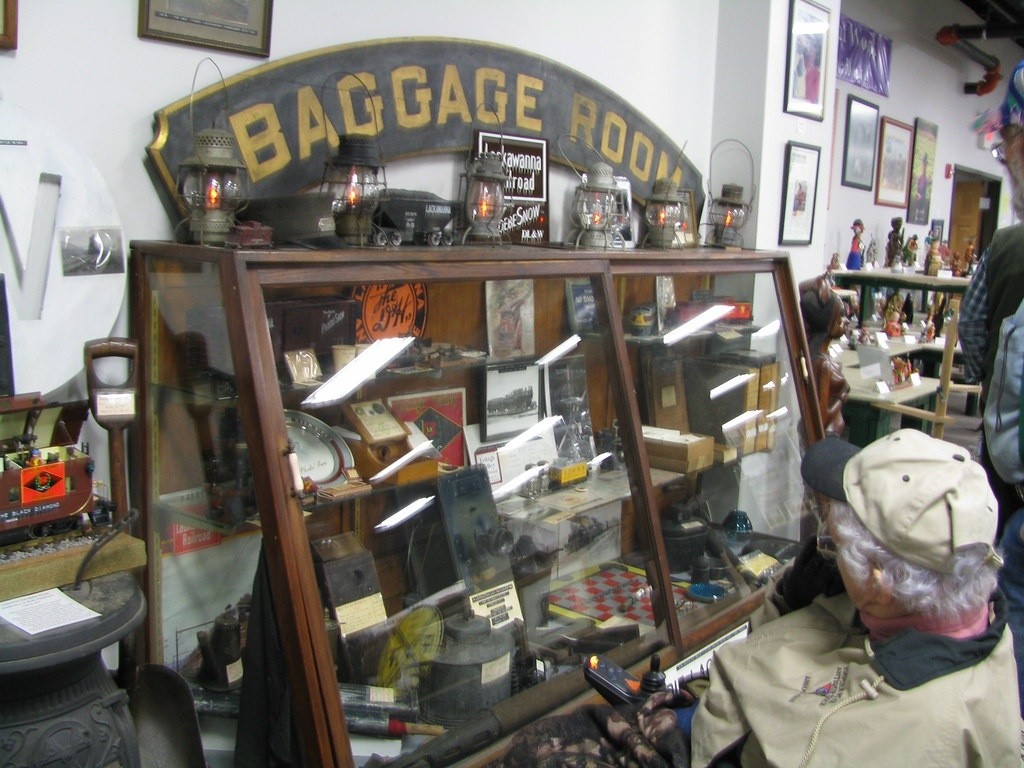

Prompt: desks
xmin=0 ymin=571 xmax=148 ymax=768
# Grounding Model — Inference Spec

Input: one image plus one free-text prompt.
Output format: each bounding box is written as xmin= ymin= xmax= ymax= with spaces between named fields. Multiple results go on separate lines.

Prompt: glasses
xmin=990 ymin=127 xmax=1024 ymax=164
xmin=816 ymin=520 xmax=841 ymax=561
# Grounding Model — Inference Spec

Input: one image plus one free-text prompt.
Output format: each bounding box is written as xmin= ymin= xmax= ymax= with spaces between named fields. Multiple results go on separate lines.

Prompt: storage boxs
xmin=642 ymin=361 xmax=783 ymax=473
xmin=187 ymin=293 xmax=358 ymax=373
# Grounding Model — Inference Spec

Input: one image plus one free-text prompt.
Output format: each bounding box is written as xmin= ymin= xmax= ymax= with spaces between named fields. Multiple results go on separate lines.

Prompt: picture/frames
xmin=931 ymin=219 xmax=944 ymax=246
xmin=778 ymin=140 xmax=821 ymax=246
xmin=840 ymin=92 xmax=915 ymax=210
xmin=672 ymin=188 xmax=699 ymax=249
xmin=480 ymin=362 xmax=542 ymax=442
xmin=784 ymin=0 xmax=833 ymax=122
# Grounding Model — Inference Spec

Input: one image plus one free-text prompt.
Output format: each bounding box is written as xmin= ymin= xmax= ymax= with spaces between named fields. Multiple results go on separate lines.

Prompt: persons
xmin=480 ymin=60 xmax=1023 ymax=768
xmin=795 ymin=274 xmax=851 ymax=434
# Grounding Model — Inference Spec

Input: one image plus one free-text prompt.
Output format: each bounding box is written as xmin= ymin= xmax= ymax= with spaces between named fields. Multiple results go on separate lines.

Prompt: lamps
xmin=639 ymin=141 xmax=688 ymax=252
xmin=454 ymin=102 xmax=511 ymax=251
xmin=174 ymin=57 xmax=253 ymax=247
xmin=318 ymin=71 xmax=389 ymax=249
xmin=703 ymin=138 xmax=757 ymax=249
xmin=556 ymin=133 xmax=626 ymax=250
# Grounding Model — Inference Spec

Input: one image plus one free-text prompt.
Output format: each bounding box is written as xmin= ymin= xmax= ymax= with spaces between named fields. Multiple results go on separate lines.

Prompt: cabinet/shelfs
xmin=131 ymin=238 xmax=821 ymax=768
xmin=832 ymin=268 xmax=984 ymax=447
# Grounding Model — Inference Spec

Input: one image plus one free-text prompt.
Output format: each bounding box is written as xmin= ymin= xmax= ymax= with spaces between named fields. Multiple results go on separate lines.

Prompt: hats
xmin=800 ymin=427 xmax=999 ymax=575
xmin=1000 ymin=57 xmax=1024 ymax=127
